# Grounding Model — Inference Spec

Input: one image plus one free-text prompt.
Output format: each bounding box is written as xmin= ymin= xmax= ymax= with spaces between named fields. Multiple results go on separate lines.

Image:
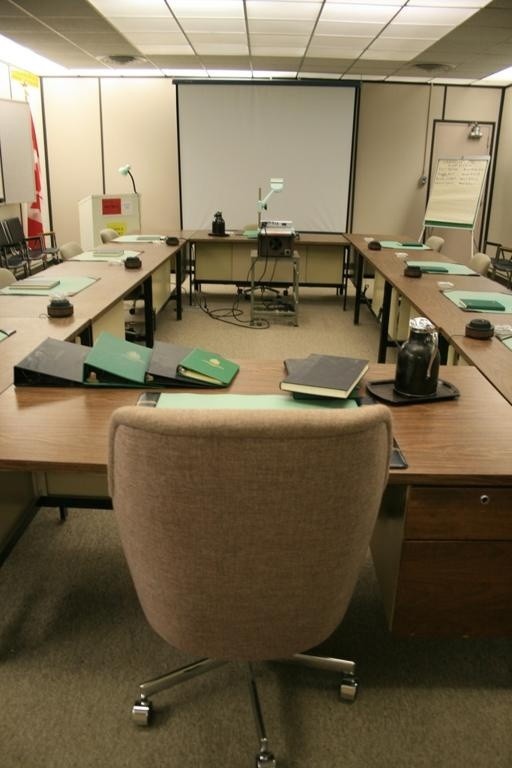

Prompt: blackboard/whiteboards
xmin=423 ymin=155 xmax=490 ymax=231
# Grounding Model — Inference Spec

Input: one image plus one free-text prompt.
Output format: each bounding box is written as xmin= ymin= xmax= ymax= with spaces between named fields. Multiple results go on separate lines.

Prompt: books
xmin=175 ymin=344 xmax=242 ymax=388
xmin=458 ymin=297 xmax=507 ymax=313
xmin=91 ymin=247 xmax=125 ymax=258
xmin=282 ymin=357 xmax=368 ymax=403
xmin=280 ymin=352 xmax=372 ymax=400
xmin=7 ymin=277 xmax=60 ymax=290
xmin=136 ymin=235 xmax=161 ymax=242
xmin=417 ymin=264 xmax=450 ymax=274
xmin=395 ymin=241 xmax=424 ymax=247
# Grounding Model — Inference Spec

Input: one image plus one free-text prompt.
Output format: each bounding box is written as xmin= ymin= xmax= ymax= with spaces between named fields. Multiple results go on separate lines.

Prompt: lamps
xmin=118 ymin=163 xmax=140 ymax=197
xmin=242 ymin=177 xmax=285 ymax=239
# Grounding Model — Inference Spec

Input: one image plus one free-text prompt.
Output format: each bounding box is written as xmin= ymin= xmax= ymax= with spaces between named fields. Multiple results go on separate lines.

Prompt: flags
xmin=24 ymin=98 xmax=51 ymax=263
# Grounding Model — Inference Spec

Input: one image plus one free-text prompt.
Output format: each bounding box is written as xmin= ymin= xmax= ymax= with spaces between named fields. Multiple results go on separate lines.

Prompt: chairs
xmin=1 ymin=216 xmax=60 ymax=280
xmin=102 ymin=396 xmax=400 ymax=767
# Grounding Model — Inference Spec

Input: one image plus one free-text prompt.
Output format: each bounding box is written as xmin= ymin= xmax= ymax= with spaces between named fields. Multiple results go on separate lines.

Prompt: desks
xmin=0 ymin=228 xmax=512 ymax=639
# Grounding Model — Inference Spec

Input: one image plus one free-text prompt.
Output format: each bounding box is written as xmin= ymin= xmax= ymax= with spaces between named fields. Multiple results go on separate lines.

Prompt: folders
xmin=14 ymin=332 xmax=220 ymax=388
